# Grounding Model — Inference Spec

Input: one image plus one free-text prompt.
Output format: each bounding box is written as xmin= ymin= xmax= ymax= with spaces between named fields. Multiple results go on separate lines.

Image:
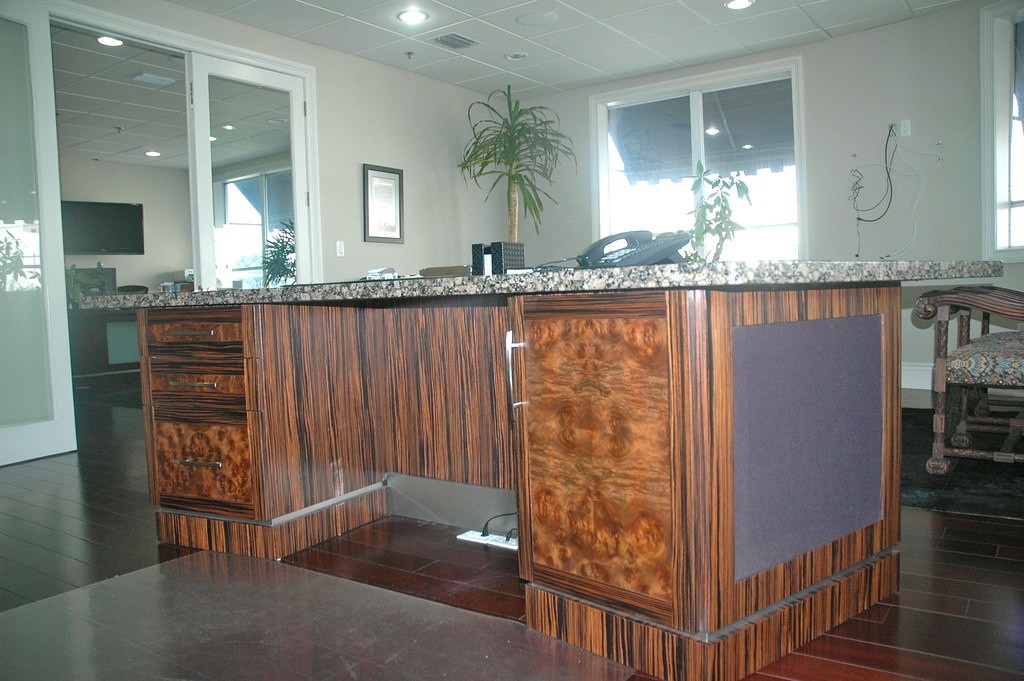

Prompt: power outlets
xmin=900 ymin=120 xmax=912 ymax=137
xmin=887 ymin=121 xmax=898 ymax=137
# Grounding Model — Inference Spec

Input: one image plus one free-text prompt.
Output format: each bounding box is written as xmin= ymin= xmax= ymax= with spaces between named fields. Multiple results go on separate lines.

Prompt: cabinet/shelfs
xmin=137 ymin=304 xmax=390 ymax=561
xmin=507 ymin=282 xmax=902 ymax=681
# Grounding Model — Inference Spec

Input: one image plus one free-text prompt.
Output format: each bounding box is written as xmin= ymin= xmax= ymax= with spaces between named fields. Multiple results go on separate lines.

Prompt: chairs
xmin=915 ymin=286 xmax=1024 ymax=475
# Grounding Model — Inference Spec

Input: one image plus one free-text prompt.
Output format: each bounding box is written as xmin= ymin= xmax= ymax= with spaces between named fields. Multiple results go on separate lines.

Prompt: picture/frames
xmin=363 ymin=164 xmax=404 ymax=244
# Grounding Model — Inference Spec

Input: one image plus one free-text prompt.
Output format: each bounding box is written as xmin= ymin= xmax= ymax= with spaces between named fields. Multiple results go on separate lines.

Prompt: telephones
xmin=571 ymin=229 xmax=691 ymax=269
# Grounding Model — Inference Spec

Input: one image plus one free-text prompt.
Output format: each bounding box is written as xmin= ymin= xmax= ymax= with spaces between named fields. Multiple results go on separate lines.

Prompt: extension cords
xmin=455 ymin=530 xmax=519 ymax=551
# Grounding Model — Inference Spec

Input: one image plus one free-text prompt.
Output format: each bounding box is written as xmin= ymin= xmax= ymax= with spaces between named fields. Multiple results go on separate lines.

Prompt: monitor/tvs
xmin=61 ymin=201 xmax=144 ymax=256
